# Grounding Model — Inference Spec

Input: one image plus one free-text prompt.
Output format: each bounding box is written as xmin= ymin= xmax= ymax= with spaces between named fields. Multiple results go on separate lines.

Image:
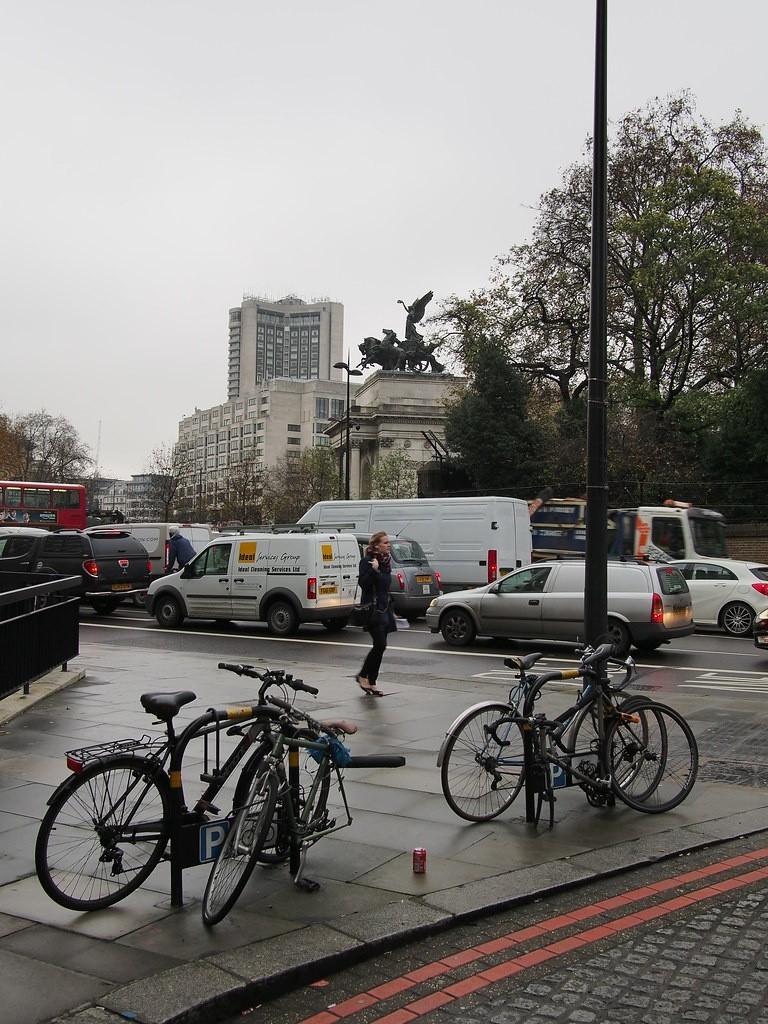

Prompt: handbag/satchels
xmin=349 ymin=600 xmax=377 ymax=625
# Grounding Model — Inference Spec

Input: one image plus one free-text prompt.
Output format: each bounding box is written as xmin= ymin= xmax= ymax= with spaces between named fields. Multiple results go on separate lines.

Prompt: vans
xmin=146 ymin=523 xmax=362 ymax=638
xmin=81 ymin=522 xmax=214 ymax=609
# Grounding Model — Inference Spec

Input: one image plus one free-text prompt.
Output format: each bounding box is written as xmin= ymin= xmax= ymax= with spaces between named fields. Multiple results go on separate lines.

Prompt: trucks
xmin=527 ymin=497 xmax=728 ymax=570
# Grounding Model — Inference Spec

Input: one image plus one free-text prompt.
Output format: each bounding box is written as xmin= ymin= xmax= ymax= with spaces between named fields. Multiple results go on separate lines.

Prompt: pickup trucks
xmin=0 ymin=528 xmax=152 ymax=614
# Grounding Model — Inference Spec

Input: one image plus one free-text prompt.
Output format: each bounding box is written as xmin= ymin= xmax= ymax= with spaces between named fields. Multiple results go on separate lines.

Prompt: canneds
xmin=413 ymin=847 xmax=427 ymax=873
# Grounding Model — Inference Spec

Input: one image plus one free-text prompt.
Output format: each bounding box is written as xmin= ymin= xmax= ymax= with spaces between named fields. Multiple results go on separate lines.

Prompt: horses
xmin=356 ymin=329 xmax=406 ymax=370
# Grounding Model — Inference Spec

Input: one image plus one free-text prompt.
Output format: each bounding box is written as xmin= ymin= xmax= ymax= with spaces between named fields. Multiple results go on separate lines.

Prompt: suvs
xmin=424 ymin=556 xmax=695 ymax=659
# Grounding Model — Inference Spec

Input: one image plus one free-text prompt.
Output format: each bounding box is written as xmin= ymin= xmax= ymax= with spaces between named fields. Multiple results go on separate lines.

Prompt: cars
xmin=753 ymin=607 xmax=768 ymax=651
xmin=668 ymin=558 xmax=768 ymax=638
xmin=0 ymin=526 xmax=48 ymax=558
xmin=354 ymin=534 xmax=443 ymax=627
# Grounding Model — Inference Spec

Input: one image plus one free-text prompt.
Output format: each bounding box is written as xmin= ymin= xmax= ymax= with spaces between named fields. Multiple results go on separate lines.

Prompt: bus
xmin=0 ymin=480 xmax=86 ymax=532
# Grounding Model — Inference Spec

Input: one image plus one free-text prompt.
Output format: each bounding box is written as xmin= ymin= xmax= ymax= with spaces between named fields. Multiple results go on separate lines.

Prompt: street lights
xmin=333 ymin=354 xmax=363 ymax=500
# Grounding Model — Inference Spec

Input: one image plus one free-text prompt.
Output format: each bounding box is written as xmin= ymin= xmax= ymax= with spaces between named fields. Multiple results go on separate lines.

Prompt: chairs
xmin=695 ymin=567 xmax=719 ymax=579
xmin=9 ymin=501 xmax=80 ymax=509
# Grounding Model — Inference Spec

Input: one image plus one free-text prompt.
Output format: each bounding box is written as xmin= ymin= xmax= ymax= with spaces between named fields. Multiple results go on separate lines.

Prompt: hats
xmin=20 ymin=510 xmax=32 ymax=514
xmin=168 ymin=526 xmax=180 ymax=534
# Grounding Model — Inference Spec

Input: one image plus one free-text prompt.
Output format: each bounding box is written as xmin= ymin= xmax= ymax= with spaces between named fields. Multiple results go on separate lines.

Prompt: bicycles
xmin=437 ymin=642 xmax=699 ymax=828
xmin=33 ymin=659 xmax=406 ymax=929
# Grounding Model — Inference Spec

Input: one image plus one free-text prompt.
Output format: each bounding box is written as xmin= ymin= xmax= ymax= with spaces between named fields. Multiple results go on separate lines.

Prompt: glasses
xmin=0 ymin=511 xmax=10 ymax=515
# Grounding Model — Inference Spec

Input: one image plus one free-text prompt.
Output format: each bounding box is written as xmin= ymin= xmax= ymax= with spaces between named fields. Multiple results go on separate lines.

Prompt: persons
xmin=355 ymin=532 xmax=397 ymax=696
xmin=655 ymin=527 xmax=677 ymax=558
xmin=167 ymin=526 xmax=196 ymax=571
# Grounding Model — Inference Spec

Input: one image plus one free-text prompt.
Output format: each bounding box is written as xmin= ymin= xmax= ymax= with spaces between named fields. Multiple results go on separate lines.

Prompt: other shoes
xmin=355 ymin=673 xmax=383 ymax=696
